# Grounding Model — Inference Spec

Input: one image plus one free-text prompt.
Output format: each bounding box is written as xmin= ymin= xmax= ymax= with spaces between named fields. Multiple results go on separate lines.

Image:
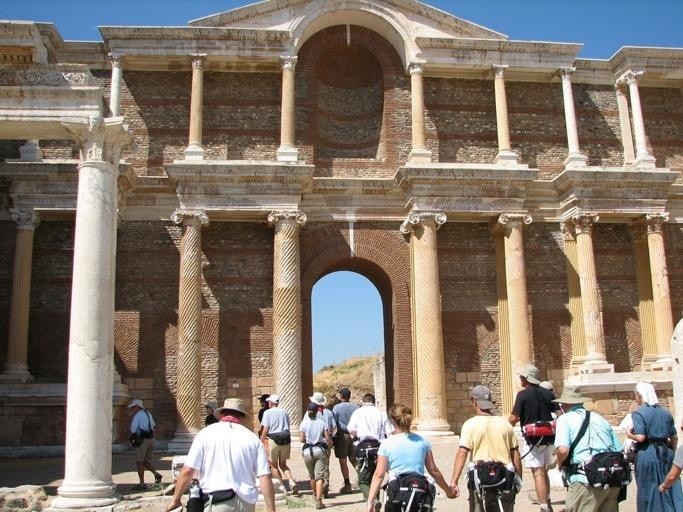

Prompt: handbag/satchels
xmin=126 ymin=433 xmax=142 ymax=448
xmin=354 ymin=439 xmax=380 ymax=461
xmin=522 ymin=419 xmax=556 ymax=438
xmin=584 ymin=451 xmax=624 ymax=488
xmin=387 ymin=471 xmax=435 ymax=510
xmin=466 ymin=461 xmax=514 ymax=495
xmin=268 ymin=431 xmax=290 ymax=444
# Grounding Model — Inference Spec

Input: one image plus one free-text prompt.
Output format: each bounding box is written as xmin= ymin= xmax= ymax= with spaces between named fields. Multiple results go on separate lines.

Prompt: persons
xmin=658 ymin=443 xmax=681 ymax=492
xmin=622 ymin=381 xmax=681 ymax=512
xmin=449 ymin=385 xmax=522 ymax=512
xmin=539 ymin=380 xmax=564 ymax=419
xmin=346 ymin=393 xmax=395 ymax=512
xmin=256 ymin=387 xmax=361 ymax=509
xmin=163 ymin=398 xmax=275 ymax=510
xmin=202 ymin=402 xmax=219 ymax=427
xmin=126 ymin=399 xmax=161 ymax=490
xmin=550 ymin=384 xmax=623 ymax=511
xmin=507 ymin=364 xmax=562 ymax=511
xmin=365 ymin=404 xmax=456 ymax=511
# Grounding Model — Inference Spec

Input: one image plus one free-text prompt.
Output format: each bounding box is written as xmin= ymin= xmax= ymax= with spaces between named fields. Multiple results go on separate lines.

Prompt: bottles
xmin=425 ymin=475 xmax=435 ymax=487
xmin=188 ymin=485 xmax=201 ymax=500
xmin=387 ymin=470 xmax=397 ymax=483
xmin=467 ymin=461 xmax=475 ymax=474
xmin=583 ymin=453 xmax=592 ymax=465
xmin=505 ymin=462 xmax=517 ymax=475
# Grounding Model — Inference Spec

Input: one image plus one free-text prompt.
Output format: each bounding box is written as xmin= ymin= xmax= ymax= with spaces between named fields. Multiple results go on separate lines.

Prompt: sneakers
xmin=373 ymin=499 xmax=381 ymax=511
xmin=289 ymin=483 xmax=329 ymax=509
xmin=340 ymin=486 xmax=350 ymax=493
xmin=131 ymin=484 xmax=146 ymax=490
xmin=154 ymin=473 xmax=161 ymax=487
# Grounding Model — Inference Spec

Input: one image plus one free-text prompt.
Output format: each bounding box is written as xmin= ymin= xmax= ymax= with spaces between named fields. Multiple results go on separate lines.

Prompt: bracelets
xmin=661 ymin=483 xmax=670 ymax=489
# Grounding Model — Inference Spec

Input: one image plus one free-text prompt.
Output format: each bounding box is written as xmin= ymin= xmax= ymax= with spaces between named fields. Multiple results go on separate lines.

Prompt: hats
xmin=257 ymin=394 xmax=268 ymax=401
xmin=204 ymin=402 xmax=217 ymax=411
xmin=516 ymin=365 xmax=593 ymax=404
xmin=470 ymin=384 xmax=494 ymax=409
xmin=127 ymin=399 xmax=144 ymax=409
xmin=308 ymin=392 xmax=326 ymax=405
xmin=265 ymin=394 xmax=279 ymax=403
xmin=213 ymin=398 xmax=250 ymax=425
